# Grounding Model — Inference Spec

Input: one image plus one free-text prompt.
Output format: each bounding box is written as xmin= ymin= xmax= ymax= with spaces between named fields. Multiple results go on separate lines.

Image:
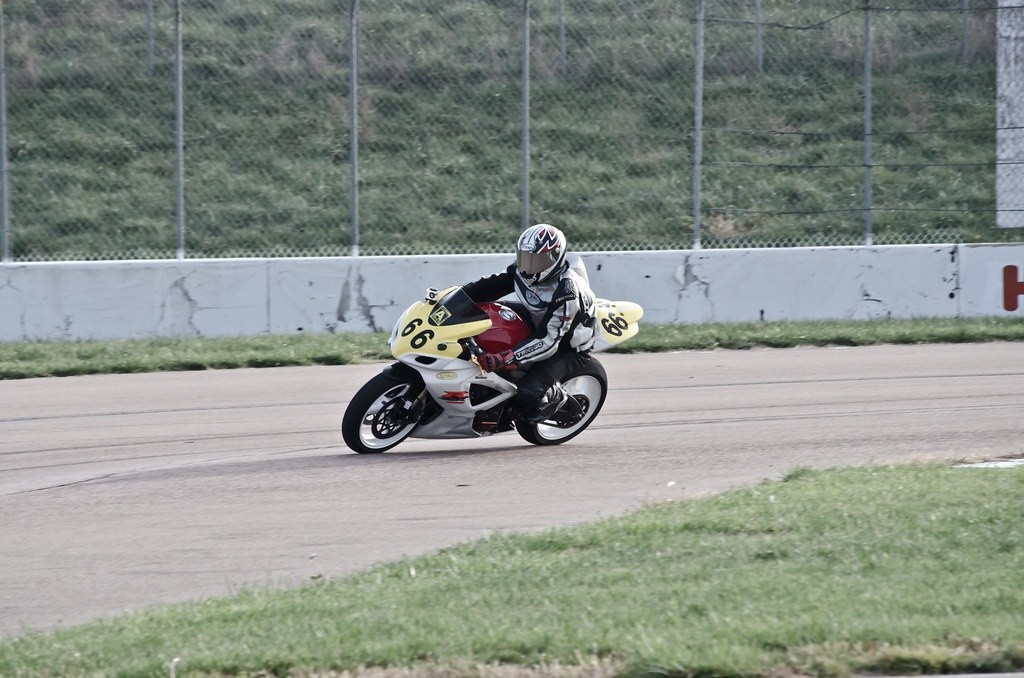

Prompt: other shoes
xmin=519 ymin=384 xmax=568 ymax=425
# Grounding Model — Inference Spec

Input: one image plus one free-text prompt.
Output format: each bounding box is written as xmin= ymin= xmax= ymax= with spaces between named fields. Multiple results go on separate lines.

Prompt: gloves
xmin=448 ymin=283 xmax=481 ymax=313
xmin=477 ymin=350 xmax=517 ymax=373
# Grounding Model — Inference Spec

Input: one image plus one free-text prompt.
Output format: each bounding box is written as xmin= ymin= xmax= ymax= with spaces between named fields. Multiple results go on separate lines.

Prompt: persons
xmin=465 ymin=224 xmax=598 ymax=422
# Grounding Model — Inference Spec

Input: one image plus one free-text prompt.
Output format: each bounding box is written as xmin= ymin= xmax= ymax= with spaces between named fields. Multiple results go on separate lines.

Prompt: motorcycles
xmin=338 ymin=283 xmax=644 ymax=455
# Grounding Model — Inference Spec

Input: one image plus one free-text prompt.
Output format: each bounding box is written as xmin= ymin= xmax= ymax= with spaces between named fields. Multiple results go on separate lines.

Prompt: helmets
xmin=516 ymin=224 xmax=567 ymax=287
